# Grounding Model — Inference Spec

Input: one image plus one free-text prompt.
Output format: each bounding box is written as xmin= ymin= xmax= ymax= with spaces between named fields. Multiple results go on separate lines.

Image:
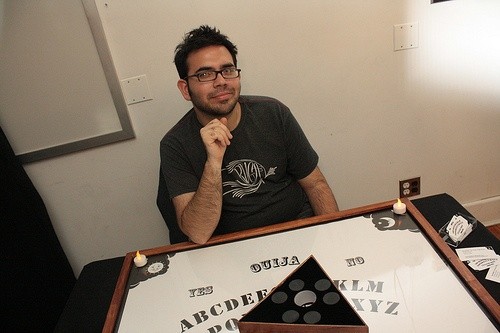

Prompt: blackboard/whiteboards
xmin=0 ymin=0 xmax=136 ymax=165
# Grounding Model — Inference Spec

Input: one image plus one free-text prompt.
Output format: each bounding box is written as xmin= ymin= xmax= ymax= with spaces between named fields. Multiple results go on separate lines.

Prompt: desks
xmin=53 ymin=192 xmax=500 ymax=333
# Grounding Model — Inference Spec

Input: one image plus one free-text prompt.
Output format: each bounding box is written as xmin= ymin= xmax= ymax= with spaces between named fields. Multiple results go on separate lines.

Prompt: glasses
xmin=184 ymin=68 xmax=241 ymax=82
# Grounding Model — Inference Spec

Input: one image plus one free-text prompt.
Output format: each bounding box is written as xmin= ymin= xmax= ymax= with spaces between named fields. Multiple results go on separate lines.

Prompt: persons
xmin=160 ymin=25 xmax=338 ymax=244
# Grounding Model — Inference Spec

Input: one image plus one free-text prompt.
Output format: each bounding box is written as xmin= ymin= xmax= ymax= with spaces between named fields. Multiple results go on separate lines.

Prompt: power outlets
xmin=400 ymin=177 xmax=421 ymax=199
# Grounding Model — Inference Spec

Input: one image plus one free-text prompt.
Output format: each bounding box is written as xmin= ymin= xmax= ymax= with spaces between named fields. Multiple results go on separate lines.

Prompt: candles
xmin=393 ymin=197 xmax=406 ymax=214
xmin=133 ymin=250 xmax=147 ymax=267
